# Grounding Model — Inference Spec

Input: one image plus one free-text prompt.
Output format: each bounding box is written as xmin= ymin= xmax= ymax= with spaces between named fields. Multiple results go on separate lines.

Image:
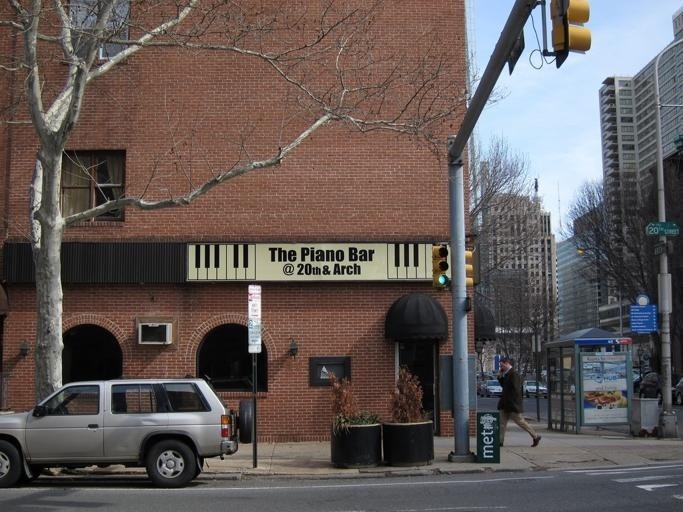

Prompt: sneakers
xmin=531 ymin=434 xmax=541 ymax=447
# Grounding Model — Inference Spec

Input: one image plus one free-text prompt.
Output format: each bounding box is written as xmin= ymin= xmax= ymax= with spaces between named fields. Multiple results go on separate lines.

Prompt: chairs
xmin=110 ymin=394 xmax=128 ymax=414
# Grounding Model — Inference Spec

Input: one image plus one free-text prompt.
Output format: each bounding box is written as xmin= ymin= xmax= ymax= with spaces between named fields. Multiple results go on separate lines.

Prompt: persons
xmin=497 ymin=359 xmax=541 ymax=447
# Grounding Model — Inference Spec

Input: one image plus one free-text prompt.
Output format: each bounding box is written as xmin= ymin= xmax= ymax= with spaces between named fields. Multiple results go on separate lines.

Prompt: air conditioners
xmin=138 ymin=322 xmax=172 ymax=345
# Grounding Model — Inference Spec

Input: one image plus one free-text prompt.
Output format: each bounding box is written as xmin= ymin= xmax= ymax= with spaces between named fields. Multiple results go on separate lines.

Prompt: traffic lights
xmin=542 ymin=0 xmax=591 ymax=68
xmin=430 ymin=243 xmax=474 ymax=288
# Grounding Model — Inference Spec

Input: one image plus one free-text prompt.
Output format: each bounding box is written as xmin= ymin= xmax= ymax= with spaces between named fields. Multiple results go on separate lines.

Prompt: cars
xmin=523 ymin=380 xmax=548 ymax=399
xmin=0 ymin=378 xmax=253 ymax=486
xmin=478 ymin=379 xmax=502 ymax=397
xmin=633 ymin=372 xmax=683 ymax=405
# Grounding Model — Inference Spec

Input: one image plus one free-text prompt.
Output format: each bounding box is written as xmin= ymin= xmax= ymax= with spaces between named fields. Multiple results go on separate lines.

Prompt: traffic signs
xmin=645 ymin=222 xmax=681 ymax=257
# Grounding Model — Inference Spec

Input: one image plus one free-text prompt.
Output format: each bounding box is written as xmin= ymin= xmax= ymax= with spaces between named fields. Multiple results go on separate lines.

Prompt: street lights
xmin=576 ymin=246 xmax=624 ymax=351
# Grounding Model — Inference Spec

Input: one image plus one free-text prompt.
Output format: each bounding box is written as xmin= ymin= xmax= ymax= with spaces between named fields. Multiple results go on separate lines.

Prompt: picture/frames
xmin=308 ymin=356 xmax=352 ymax=386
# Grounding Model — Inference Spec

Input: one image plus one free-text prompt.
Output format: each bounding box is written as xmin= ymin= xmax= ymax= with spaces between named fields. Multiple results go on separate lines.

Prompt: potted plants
xmin=328 ymin=364 xmax=435 ymax=468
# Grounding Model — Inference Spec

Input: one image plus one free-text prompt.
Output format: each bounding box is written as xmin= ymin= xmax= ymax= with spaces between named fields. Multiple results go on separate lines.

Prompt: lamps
xmin=20 ymin=340 xmax=30 ymax=357
xmin=288 ymin=340 xmax=299 ymax=358
